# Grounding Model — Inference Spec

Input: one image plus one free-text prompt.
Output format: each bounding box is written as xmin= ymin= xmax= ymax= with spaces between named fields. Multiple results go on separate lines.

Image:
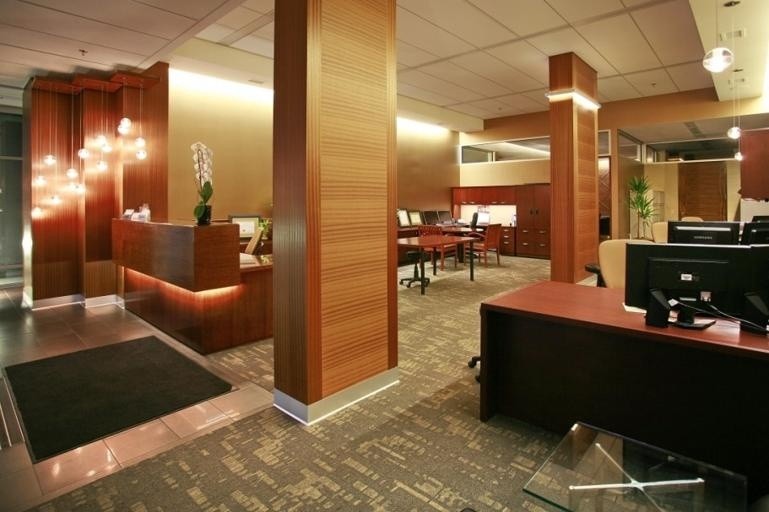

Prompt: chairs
xmin=417 ymin=224 xmax=503 ymax=271
xmin=595 ymin=215 xmax=703 ymax=288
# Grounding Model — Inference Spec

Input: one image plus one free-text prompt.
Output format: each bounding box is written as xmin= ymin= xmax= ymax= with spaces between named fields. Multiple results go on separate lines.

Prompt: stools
xmin=400 ymin=250 xmax=431 ymax=288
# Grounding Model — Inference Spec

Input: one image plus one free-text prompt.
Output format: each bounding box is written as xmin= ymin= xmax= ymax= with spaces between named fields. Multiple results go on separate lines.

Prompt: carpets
xmin=3 ymin=334 xmax=233 ymax=465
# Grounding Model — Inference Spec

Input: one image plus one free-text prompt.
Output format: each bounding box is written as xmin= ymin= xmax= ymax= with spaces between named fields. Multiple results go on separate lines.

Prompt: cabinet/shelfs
xmin=499 ymin=226 xmax=514 ymax=256
xmin=452 ymin=185 xmax=516 ymax=205
xmin=515 ymin=183 xmax=551 ymax=260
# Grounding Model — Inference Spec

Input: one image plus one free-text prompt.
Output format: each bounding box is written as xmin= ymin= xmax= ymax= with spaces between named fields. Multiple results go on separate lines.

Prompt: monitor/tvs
xmin=398 ymin=208 xmax=453 ymax=228
xmin=625 ymin=215 xmax=769 ymax=335
xmin=229 ymin=215 xmax=262 ymax=241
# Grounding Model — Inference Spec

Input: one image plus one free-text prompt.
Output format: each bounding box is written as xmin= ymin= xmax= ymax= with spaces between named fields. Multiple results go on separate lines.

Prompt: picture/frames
xmin=228 ymin=213 xmax=263 ymax=241
xmin=397 ymin=207 xmax=453 ymax=228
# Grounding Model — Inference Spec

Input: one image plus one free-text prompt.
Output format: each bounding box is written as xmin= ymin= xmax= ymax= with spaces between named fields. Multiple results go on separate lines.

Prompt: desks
xmin=481 ymin=280 xmax=769 ymax=504
xmin=397 ymin=235 xmax=480 ymax=294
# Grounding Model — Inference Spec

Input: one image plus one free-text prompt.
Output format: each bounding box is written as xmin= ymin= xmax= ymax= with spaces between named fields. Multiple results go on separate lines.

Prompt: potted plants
xmin=193 ymin=180 xmax=214 ymax=226
